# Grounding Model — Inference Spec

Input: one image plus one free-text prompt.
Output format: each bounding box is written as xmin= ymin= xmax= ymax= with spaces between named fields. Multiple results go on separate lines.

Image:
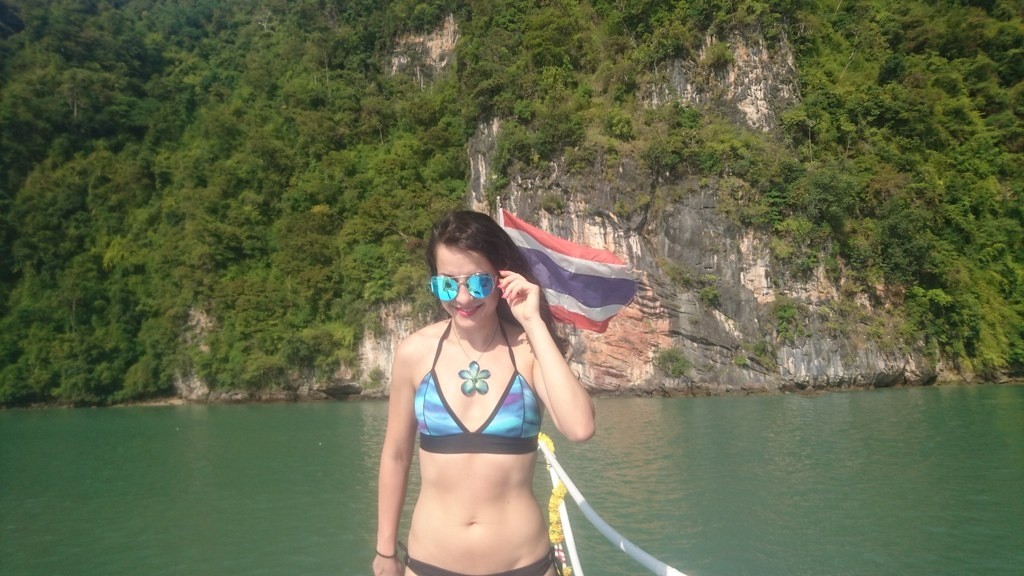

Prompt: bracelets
xmin=376 ymin=550 xmax=398 ymax=558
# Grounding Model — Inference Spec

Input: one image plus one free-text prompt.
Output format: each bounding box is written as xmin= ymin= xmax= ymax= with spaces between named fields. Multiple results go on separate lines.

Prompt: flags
xmin=499 ymin=206 xmax=635 ymax=334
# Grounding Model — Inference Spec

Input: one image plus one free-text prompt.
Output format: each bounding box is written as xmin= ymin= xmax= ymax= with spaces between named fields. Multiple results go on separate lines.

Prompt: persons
xmin=373 ymin=211 xmax=596 ymax=576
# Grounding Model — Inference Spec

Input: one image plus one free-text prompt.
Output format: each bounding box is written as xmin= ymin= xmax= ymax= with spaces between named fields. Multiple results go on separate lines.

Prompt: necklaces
xmin=450 ymin=316 xmax=499 ymax=396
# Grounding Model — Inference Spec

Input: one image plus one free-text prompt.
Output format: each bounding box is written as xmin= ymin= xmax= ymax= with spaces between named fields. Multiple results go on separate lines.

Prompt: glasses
xmin=427 ymin=272 xmax=498 ymax=302
